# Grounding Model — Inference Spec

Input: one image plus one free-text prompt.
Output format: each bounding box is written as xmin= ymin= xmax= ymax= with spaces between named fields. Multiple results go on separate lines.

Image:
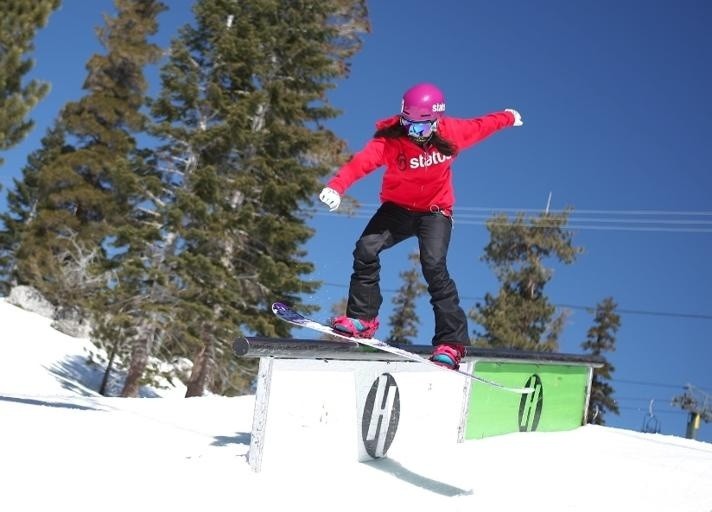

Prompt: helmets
xmin=400 ymin=83 xmax=446 ymax=122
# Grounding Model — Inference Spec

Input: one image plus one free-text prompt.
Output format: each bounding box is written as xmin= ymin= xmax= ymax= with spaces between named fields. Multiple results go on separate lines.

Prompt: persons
xmin=317 ymin=79 xmax=528 ymax=372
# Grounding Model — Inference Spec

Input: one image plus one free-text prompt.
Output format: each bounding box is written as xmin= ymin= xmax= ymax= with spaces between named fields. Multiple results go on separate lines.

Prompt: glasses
xmin=400 ymin=116 xmax=437 ymax=136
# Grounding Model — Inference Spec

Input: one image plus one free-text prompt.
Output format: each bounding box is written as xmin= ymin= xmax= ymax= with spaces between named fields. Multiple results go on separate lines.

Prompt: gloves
xmin=319 ymin=187 xmax=341 ymax=212
xmin=505 ymin=108 xmax=523 ymax=127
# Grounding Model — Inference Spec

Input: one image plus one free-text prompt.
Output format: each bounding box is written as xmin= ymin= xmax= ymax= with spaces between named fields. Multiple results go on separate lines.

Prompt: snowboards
xmin=271 ymin=302 xmax=534 ymax=393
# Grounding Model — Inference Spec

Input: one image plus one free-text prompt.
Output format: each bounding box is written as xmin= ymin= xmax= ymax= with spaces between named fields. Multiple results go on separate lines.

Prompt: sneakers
xmin=331 ymin=315 xmax=379 ymax=338
xmin=430 ymin=342 xmax=467 ymax=370
